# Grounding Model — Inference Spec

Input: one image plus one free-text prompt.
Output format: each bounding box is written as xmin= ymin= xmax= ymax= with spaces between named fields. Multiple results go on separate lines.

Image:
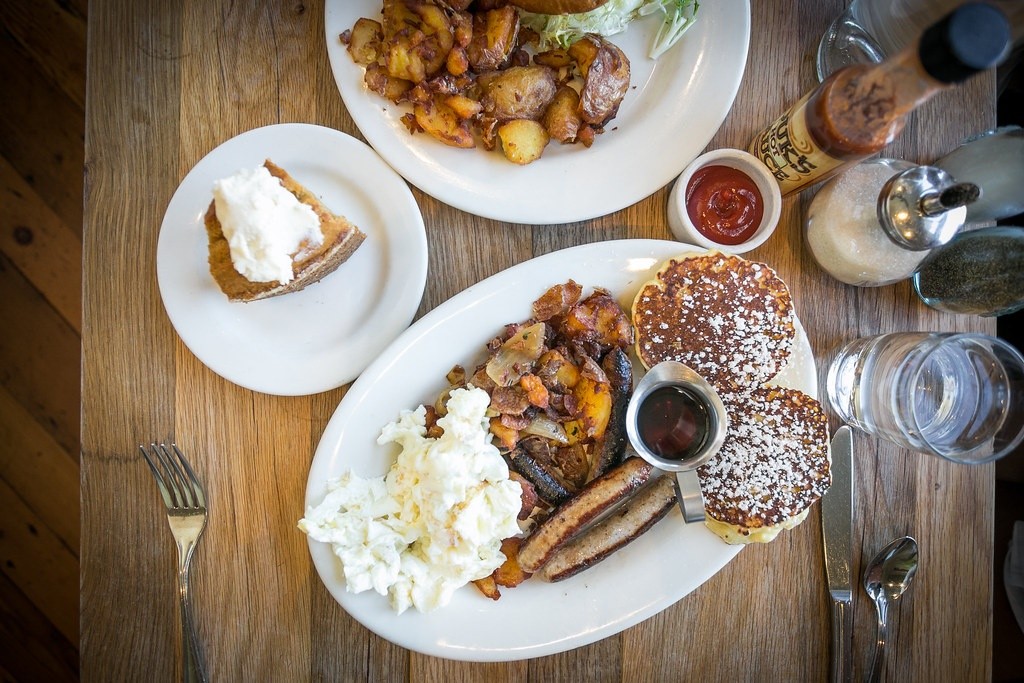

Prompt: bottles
xmin=934 ymin=126 xmax=1024 ymax=223
xmin=913 ymin=228 xmax=1024 ymax=315
xmin=747 ymin=6 xmax=1012 ymax=199
xmin=804 ymin=158 xmax=979 ymax=287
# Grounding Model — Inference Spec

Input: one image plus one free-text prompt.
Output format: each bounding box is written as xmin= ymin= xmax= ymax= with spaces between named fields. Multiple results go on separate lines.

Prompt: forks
xmin=139 ymin=443 xmax=210 ymax=683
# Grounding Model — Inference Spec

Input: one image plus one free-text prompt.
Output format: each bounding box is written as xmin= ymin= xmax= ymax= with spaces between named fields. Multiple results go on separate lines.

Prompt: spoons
xmin=863 ymin=535 xmax=919 ymax=683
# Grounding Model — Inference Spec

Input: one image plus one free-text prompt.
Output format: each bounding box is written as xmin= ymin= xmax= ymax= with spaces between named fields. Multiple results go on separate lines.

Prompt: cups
xmin=667 ymin=148 xmax=780 ymax=253
xmin=626 ymin=361 xmax=727 ymax=523
xmin=816 ymin=0 xmax=957 ymax=84
xmin=826 ymin=331 xmax=1024 ymax=465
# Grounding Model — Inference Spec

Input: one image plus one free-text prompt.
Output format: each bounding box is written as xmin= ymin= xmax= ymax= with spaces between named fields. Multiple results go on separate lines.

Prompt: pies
xmin=694 ymin=385 xmax=832 ymax=545
xmin=631 ymin=249 xmax=794 ymax=396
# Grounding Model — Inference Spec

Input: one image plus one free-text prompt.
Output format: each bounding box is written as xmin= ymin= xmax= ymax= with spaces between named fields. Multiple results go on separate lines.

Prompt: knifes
xmin=820 ymin=425 xmax=854 ymax=683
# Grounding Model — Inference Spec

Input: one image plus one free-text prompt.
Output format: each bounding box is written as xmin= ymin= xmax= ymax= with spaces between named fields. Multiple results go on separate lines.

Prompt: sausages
xmin=516 ymin=456 xmax=651 ymax=573
xmin=538 ymin=472 xmax=678 ymax=583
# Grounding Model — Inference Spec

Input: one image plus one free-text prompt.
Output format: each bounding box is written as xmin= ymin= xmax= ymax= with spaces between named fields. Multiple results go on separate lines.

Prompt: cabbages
xmin=515 ymin=0 xmax=701 ymax=59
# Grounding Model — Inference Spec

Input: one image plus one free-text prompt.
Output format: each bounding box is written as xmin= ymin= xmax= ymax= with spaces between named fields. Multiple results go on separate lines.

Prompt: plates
xmin=325 ymin=1 xmax=750 ymax=225
xmin=157 ymin=123 xmax=428 ymax=396
xmin=306 ymin=238 xmax=816 ymax=662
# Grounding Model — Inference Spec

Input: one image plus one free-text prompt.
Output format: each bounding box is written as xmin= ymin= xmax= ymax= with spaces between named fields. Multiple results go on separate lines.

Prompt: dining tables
xmin=78 ymin=0 xmax=998 ymax=683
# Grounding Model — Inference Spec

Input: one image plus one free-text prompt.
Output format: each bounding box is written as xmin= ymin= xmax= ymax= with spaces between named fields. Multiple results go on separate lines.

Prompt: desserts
xmin=203 ymin=157 xmax=367 ymax=304
